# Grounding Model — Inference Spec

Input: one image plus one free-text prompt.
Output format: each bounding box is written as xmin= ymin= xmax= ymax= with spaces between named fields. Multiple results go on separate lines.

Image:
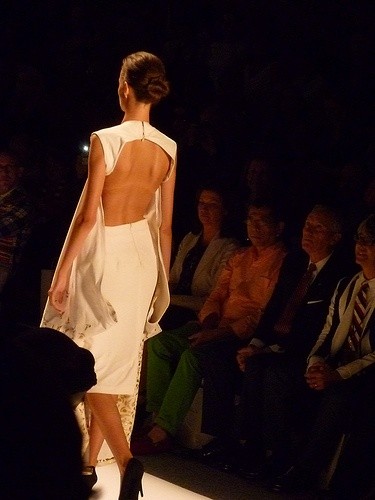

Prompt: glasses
xmin=354 ymin=232 xmax=375 ymax=247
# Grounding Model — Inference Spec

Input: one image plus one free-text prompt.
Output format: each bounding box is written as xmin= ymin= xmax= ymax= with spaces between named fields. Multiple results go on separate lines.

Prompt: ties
xmin=272 ymin=263 xmax=317 ymax=336
xmin=338 ymin=284 xmax=370 ymax=367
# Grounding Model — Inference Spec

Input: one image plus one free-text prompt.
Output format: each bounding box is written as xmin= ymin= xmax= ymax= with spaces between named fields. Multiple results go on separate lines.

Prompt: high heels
xmin=81 ymin=465 xmax=98 ymax=490
xmin=117 ymin=458 xmax=145 ymax=500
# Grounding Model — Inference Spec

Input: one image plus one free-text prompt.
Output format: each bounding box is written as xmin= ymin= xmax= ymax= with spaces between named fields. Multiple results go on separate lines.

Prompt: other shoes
xmin=198 ymin=437 xmax=226 ymax=459
xmin=133 ymin=431 xmax=173 ymax=455
xmin=240 ymin=458 xmax=273 ymax=479
xmin=273 ymin=463 xmax=303 ymax=491
xmin=220 ymin=451 xmax=242 ymax=473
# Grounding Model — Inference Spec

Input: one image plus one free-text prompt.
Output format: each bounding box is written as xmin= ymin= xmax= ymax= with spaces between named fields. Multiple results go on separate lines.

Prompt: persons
xmin=0 ymin=129 xmax=375 ymax=500
xmin=40 ymin=51 xmax=179 ymax=500
